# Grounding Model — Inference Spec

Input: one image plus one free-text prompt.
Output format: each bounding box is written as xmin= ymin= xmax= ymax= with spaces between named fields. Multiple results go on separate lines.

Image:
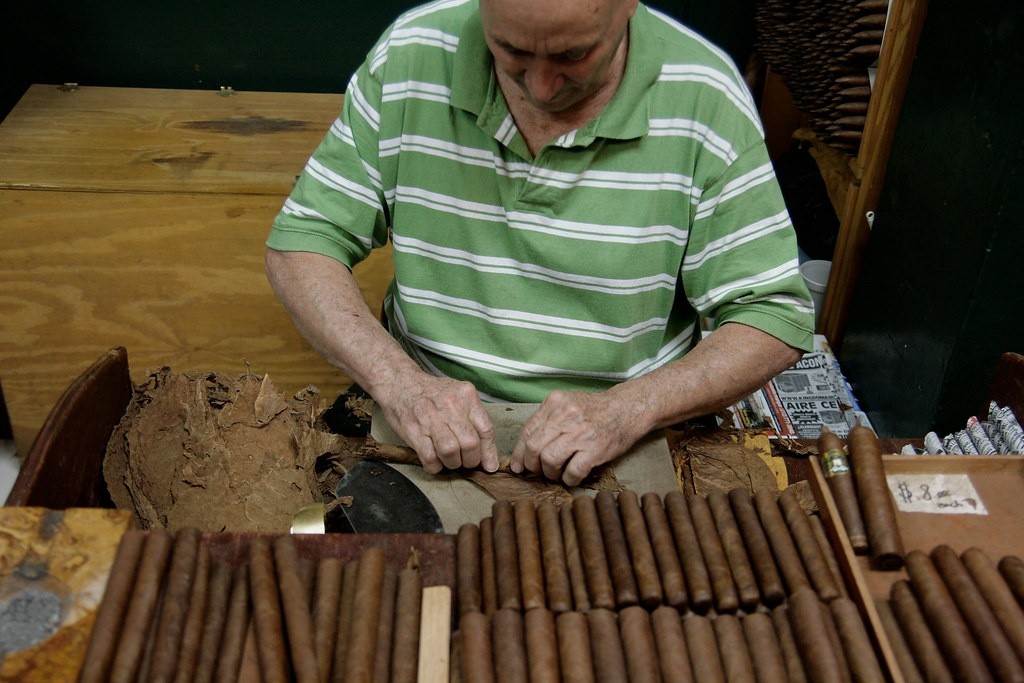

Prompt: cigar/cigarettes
xmin=458 ymin=489 xmax=1024 ymax=683
xmin=817 ymin=433 xmax=867 ymax=552
xmin=848 ymin=427 xmax=904 ymax=571
xmin=73 ymin=527 xmax=423 ymax=683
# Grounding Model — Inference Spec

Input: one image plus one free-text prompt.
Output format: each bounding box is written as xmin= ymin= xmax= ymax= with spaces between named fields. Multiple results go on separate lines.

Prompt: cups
xmin=799 ymin=259 xmax=833 ymax=328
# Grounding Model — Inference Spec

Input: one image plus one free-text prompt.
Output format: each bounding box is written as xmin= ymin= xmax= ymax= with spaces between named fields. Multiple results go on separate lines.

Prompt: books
xmin=735 ymin=334 xmax=880 ymax=447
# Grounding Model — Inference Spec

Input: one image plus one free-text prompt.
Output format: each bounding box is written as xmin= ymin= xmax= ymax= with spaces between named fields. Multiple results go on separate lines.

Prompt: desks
xmin=1 ymin=345 xmax=1024 ymax=683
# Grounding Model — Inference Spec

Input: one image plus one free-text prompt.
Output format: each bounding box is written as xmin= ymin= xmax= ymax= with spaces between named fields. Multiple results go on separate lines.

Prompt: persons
xmin=263 ymin=0 xmax=814 ymax=489
